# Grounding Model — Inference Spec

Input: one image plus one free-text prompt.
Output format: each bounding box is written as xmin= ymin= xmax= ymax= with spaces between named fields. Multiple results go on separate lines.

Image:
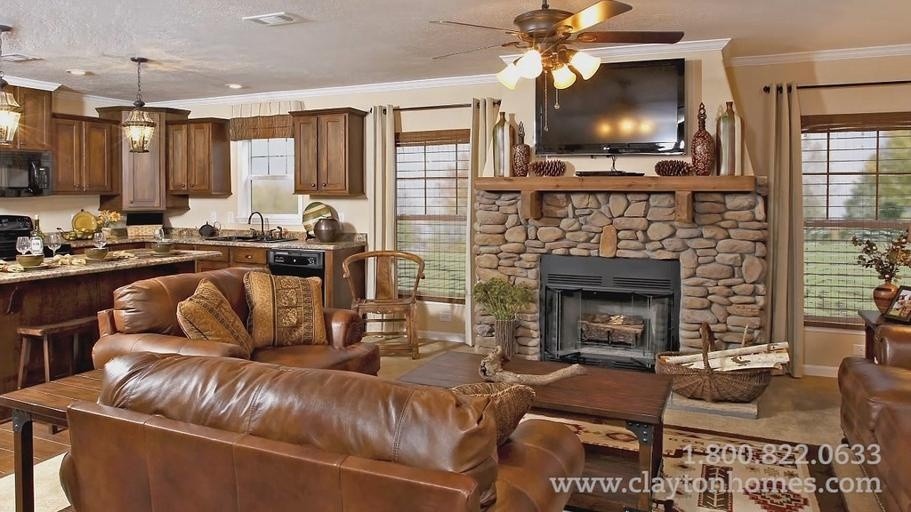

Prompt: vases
xmin=716 ymin=102 xmax=743 ymax=176
xmin=492 ymin=112 xmax=514 ymax=178
xmin=101 ymin=227 xmax=111 ymax=239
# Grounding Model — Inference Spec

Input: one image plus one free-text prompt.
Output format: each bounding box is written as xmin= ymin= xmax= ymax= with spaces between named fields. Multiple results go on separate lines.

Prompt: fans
xmin=429 ymin=0 xmax=684 ymax=131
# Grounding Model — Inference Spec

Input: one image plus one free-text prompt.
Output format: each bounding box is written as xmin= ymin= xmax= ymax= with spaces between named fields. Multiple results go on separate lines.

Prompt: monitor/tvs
xmin=535 ymin=58 xmax=688 ymax=157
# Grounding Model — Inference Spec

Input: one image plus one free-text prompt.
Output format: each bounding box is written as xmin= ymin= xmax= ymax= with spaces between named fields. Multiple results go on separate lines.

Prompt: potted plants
xmin=850 ymin=229 xmax=911 ymax=316
xmin=474 ymin=279 xmax=535 ymax=360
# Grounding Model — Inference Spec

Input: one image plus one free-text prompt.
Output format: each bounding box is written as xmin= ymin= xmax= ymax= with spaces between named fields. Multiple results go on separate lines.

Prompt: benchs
xmin=17 ymin=314 xmax=98 ymax=434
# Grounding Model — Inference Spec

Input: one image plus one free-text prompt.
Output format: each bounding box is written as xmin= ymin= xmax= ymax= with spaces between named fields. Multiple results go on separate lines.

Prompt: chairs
xmin=340 ymin=250 xmax=426 ymax=360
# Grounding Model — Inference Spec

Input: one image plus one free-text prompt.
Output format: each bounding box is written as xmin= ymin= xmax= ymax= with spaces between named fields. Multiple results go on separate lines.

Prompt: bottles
xmin=31 ymin=214 xmax=43 ymax=238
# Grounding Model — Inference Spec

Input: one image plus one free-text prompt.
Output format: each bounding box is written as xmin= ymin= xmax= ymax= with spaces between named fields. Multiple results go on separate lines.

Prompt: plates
xmin=71 ymin=212 xmax=98 ymax=231
xmin=79 ymin=256 xmax=119 ymax=262
xmin=9 ymin=263 xmax=49 ymax=270
xmin=146 ymin=250 xmax=180 ymax=257
xmin=301 ymin=201 xmax=331 ymax=237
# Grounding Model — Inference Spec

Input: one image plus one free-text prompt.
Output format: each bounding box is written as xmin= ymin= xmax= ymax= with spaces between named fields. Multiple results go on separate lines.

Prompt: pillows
xmin=178 ymin=271 xmax=328 ymax=361
xmin=448 ymin=378 xmax=535 ymax=447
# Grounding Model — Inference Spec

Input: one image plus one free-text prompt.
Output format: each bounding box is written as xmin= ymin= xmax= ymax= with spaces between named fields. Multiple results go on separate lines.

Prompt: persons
xmin=899 ymin=296 xmax=911 ymax=317
xmin=893 ymin=295 xmax=906 ymax=310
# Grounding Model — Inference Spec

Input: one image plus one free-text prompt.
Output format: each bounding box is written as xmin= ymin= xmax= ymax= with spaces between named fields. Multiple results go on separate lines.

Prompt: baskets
xmin=654 ymin=321 xmax=773 ymax=404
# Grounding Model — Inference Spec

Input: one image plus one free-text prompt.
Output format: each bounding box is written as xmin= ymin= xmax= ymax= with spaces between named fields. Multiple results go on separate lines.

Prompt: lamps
xmin=1 ymin=25 xmax=23 ymax=146
xmin=495 ymin=40 xmax=601 ymax=131
xmin=121 ymin=56 xmax=156 ymax=154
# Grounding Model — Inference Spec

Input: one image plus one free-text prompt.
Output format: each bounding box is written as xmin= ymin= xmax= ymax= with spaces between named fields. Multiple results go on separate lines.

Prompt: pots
xmin=56 ymin=228 xmax=89 ymax=241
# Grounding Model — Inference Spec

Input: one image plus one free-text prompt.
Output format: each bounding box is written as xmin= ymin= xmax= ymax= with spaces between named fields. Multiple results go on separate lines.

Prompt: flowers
xmin=98 ymin=209 xmax=122 ymax=227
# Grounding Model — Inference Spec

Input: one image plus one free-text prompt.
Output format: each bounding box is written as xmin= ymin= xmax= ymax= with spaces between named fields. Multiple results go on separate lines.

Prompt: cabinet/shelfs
xmin=0 ymin=84 xmax=232 ymax=213
xmin=194 ymin=245 xmax=267 ymax=275
xmin=288 ymin=108 xmax=366 ymax=199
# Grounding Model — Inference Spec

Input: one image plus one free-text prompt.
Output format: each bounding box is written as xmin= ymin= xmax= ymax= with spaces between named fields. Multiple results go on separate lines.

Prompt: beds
xmin=858 ymin=310 xmax=906 ymax=360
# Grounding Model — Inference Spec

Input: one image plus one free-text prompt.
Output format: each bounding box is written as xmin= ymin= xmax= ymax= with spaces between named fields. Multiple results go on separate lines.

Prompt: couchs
xmin=92 ymin=266 xmax=380 ymax=381
xmin=838 ymin=326 xmax=911 ymax=512
xmin=61 ymin=352 xmax=584 ymax=512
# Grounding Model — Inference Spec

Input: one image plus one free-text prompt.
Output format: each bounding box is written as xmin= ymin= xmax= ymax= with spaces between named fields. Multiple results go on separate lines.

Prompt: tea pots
xmin=195 ymin=221 xmax=221 ymax=236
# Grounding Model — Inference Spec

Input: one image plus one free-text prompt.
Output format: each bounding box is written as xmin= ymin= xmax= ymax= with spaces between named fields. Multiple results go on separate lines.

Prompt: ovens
xmin=269 ymin=265 xmax=324 ymax=308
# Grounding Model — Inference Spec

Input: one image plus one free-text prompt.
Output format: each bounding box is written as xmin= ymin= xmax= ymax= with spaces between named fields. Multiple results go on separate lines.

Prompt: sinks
xmin=206 ymin=236 xmax=257 ymax=242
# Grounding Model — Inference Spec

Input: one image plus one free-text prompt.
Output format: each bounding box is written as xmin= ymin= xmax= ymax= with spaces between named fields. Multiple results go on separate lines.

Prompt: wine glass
xmin=30 ymin=237 xmax=43 ymax=256
xmin=47 ymin=234 xmax=62 ymax=257
xmin=93 ymin=233 xmax=107 ymax=248
xmin=153 ymin=228 xmax=165 ymax=243
xmin=15 ymin=236 xmax=31 ymax=254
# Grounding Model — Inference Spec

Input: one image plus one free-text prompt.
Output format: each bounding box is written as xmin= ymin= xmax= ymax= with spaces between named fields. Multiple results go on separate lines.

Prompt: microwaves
xmin=0 ymin=150 xmax=53 ymax=197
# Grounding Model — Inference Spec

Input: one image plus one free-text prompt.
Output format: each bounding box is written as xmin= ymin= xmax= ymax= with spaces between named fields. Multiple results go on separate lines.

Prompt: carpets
xmin=511 ymin=409 xmax=849 ymax=511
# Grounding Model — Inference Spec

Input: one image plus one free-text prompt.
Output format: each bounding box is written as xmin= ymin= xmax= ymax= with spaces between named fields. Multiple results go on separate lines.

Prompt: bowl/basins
xmin=84 ymin=247 xmax=109 ymax=260
xmin=152 ymin=243 xmax=173 ymax=252
xmin=16 ymin=254 xmax=45 ymax=266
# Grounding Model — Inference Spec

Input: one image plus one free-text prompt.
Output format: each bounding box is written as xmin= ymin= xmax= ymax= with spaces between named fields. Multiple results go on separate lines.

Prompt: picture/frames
xmin=882 ymin=285 xmax=911 ymax=325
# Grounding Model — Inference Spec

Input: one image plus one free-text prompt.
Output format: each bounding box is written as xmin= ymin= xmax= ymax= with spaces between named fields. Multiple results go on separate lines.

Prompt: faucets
xmin=248 ymin=211 xmax=263 ymax=235
